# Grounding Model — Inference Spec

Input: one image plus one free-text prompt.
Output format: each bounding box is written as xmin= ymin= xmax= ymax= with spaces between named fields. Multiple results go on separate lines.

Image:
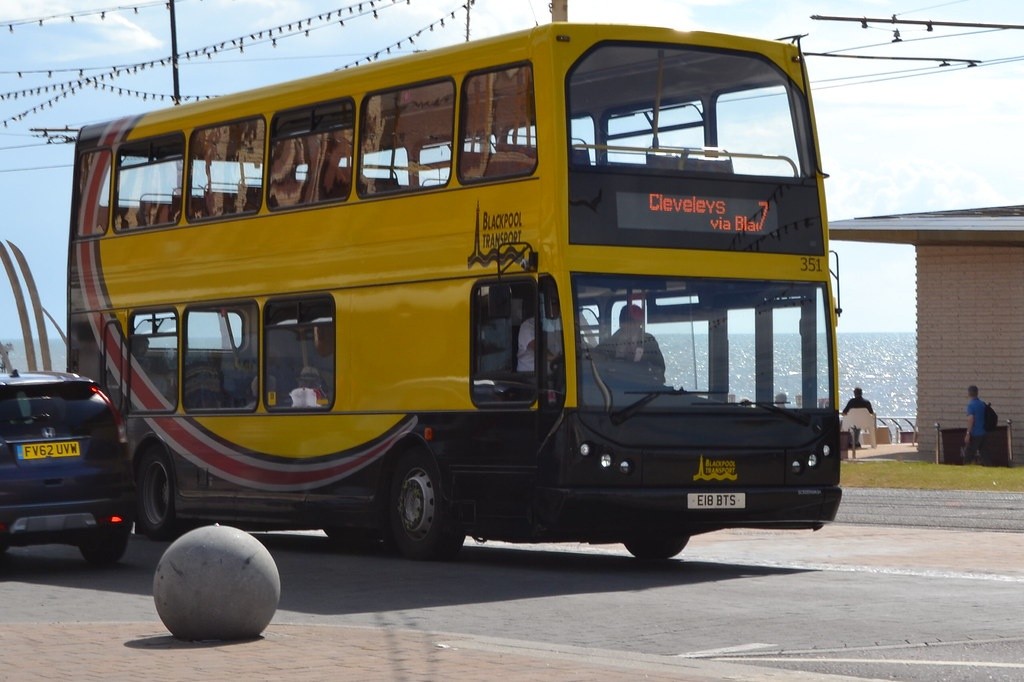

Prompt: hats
xmin=621 ymin=305 xmax=643 ymax=320
xmin=298 ymin=366 xmax=319 ymax=380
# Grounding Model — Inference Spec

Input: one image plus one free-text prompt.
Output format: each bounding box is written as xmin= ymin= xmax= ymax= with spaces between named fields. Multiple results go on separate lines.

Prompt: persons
xmin=289 ymin=368 xmax=328 ymax=409
xmin=515 ymin=281 xmax=598 ymax=409
xmin=610 ymin=304 xmax=665 ymax=384
xmin=963 ymin=385 xmax=992 ymax=466
xmin=842 ymin=387 xmax=874 ymax=447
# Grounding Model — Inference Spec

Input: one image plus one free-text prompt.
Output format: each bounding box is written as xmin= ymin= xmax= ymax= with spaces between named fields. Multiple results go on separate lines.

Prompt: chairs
xmin=840 ymin=408 xmax=876 ymax=449
xmin=132 ymin=355 xmax=331 ymax=410
xmin=109 ymin=134 xmax=593 ymax=233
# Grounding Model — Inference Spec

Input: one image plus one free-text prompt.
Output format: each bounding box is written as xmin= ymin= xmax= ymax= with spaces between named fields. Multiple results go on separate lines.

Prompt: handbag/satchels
xmin=983 ymin=402 xmax=997 ymax=431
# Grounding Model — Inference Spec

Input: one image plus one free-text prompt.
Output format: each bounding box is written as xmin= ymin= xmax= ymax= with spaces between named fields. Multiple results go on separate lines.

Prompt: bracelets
xmin=967 ymin=432 xmax=970 ymax=434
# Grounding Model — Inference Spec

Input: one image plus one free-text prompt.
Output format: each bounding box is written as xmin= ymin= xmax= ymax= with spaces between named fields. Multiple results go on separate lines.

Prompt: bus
xmin=66 ymin=19 xmax=844 ymax=563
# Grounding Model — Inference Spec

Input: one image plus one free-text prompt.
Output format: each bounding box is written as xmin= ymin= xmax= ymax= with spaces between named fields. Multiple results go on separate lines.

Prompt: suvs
xmin=0 ymin=369 xmax=138 ymax=564
